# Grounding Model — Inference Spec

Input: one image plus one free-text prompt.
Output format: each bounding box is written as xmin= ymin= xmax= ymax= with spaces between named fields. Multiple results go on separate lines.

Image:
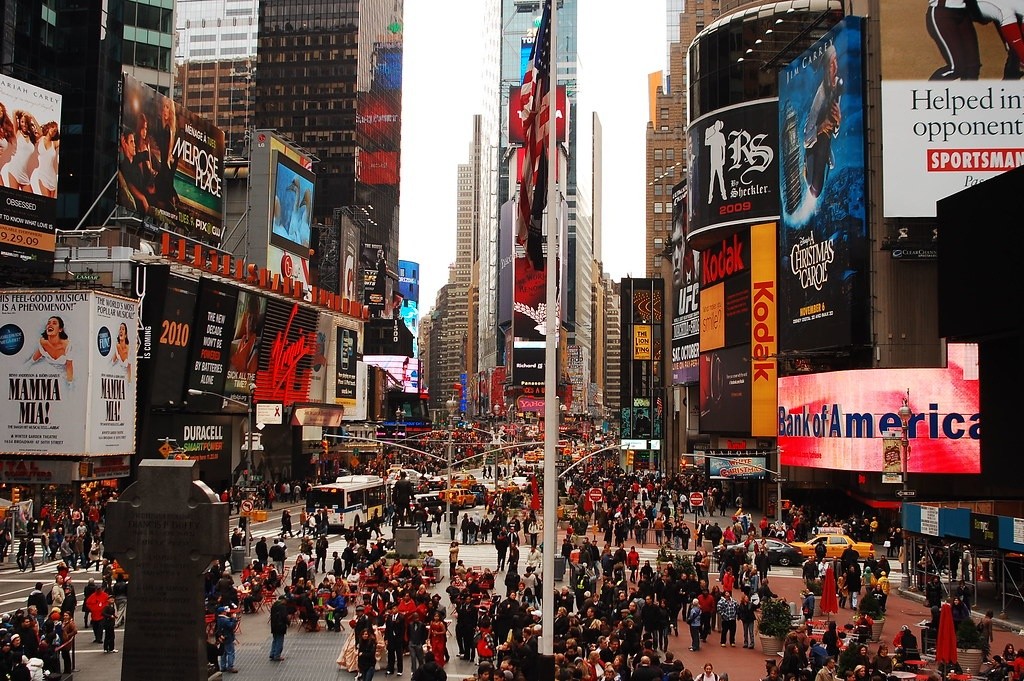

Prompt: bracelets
xmin=111 ymin=615 xmax=114 ymax=618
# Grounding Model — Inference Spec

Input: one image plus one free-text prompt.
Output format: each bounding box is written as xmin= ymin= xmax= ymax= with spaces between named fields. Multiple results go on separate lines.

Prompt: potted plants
xmin=399 ymin=555 xmax=408 ymax=565
xmin=385 ymin=553 xmax=394 ymax=566
xmin=854 ymin=595 xmax=886 ymax=641
xmin=956 ymin=619 xmax=990 ymax=676
xmin=755 ymin=600 xmax=792 ymax=655
xmin=410 ymin=555 xmax=417 ymax=565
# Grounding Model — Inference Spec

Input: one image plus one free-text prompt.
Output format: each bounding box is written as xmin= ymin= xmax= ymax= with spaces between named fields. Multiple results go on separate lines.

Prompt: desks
xmin=887 ymin=653 xmax=900 ymax=657
xmin=206 ymin=618 xmax=214 ymax=630
xmin=951 ymin=675 xmax=967 ymax=681
xmin=888 ymin=671 xmax=917 ymax=681
xmin=904 ymin=660 xmax=927 ymax=669
xmin=809 ymin=635 xmax=823 ymax=642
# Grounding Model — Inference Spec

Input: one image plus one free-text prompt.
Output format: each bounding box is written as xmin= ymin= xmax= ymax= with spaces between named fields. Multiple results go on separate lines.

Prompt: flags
xmin=519 ymin=0 xmax=551 ymax=273
xmin=657 ymin=393 xmax=662 ymax=419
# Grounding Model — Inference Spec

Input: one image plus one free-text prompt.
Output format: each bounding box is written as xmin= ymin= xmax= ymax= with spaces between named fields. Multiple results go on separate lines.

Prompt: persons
xmin=24 ymin=316 xmax=75 ymax=386
xmin=234 ymin=296 xmax=261 ymax=374
xmin=118 ymin=95 xmax=184 ymax=227
xmin=275 ymin=177 xmax=311 ymax=247
xmin=634 ymin=409 xmax=650 ymax=436
xmin=0 ymin=424 xmax=1024 ymax=681
xmin=671 ymin=205 xmax=685 ymax=299
xmin=106 ymin=322 xmax=133 ymax=384
xmin=0 ymin=103 xmax=60 ymax=198
xmin=803 ymin=45 xmax=842 ymax=187
xmin=369 ymin=250 xmax=387 ymax=297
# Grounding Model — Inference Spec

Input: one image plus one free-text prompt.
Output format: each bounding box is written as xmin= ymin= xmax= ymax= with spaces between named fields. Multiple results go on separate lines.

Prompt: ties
xmin=394 ymin=614 xmax=395 ymax=621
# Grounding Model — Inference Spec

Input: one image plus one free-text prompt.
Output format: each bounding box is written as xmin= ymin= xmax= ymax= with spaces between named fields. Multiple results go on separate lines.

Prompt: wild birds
xmin=272 ymin=176 xmax=311 ymax=249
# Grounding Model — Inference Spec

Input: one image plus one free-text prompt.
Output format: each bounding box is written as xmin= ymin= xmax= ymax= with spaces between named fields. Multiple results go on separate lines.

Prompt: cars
xmin=452 ymin=472 xmax=477 ymax=487
xmin=509 ymin=476 xmax=531 ymax=490
xmin=789 ymin=533 xmax=876 ymax=565
xmin=712 ymin=536 xmax=801 ymax=567
xmin=428 ymin=476 xmax=447 ymax=490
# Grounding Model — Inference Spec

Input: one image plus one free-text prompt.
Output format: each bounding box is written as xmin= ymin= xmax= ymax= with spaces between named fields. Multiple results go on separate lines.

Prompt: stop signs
xmin=590 ymin=489 xmax=602 ymax=502
xmin=690 ymin=492 xmax=702 ymax=505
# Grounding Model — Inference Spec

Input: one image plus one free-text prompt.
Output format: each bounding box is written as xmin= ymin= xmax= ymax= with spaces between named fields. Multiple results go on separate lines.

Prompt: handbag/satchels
xmin=687 ymin=617 xmax=692 ymax=625
xmin=91 ymin=544 xmax=98 ymax=555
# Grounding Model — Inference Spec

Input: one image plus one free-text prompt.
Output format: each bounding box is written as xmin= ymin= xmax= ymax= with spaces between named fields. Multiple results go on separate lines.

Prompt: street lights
xmin=395 ymin=406 xmax=401 ymax=463
xmin=188 ymin=388 xmax=258 ymax=557
xmin=882 ymin=398 xmax=912 ymax=589
xmin=494 ymin=401 xmax=500 ymax=489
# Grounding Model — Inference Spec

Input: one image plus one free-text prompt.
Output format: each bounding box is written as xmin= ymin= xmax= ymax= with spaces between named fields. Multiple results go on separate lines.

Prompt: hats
xmin=217 ymin=607 xmax=227 ymax=615
xmin=854 ymin=664 xmax=864 ymax=672
xmin=278 ymin=595 xmax=286 ymax=599
xmin=50 ymin=577 xmax=114 ymax=622
xmin=531 ymin=610 xmax=542 ymax=616
xmin=10 ymin=634 xmax=19 ymax=641
xmin=867 ymin=569 xmax=886 ymax=579
xmin=693 ymin=599 xmax=699 ymax=605
xmin=2 ymin=614 xmax=10 ymax=620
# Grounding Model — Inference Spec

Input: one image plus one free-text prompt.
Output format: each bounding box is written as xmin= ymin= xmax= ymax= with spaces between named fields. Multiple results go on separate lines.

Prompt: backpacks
xmin=46 ymin=585 xmax=61 ymax=605
xmin=976 ymin=618 xmax=985 ymax=634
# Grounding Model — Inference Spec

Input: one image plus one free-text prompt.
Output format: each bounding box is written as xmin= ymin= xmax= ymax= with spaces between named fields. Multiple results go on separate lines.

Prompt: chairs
xmin=786 ymin=614 xmax=1016 ymax=681
xmin=206 ymin=566 xmax=501 ymax=643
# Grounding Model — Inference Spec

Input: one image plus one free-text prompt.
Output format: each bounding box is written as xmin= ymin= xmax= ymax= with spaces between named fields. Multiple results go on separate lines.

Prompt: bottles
xmin=507 ymin=629 xmax=512 ymax=642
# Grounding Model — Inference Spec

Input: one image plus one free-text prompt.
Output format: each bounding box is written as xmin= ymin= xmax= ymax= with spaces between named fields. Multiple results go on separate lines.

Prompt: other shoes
xmin=104 ymin=649 xmax=119 ymax=653
xmin=688 ymin=643 xmax=753 ymax=652
xmin=221 ymin=666 xmax=238 ymax=673
xmin=397 ymin=672 xmax=403 ymax=676
xmin=385 ymin=671 xmax=391 ymax=675
xmin=269 ymin=656 xmax=285 ymax=661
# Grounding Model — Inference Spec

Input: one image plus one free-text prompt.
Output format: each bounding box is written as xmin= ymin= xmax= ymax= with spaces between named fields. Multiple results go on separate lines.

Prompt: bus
xmin=306 ymin=474 xmax=386 ymax=535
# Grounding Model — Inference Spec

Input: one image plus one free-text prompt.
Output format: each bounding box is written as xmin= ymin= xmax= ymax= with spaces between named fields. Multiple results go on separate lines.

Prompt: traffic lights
xmin=448 ymin=491 xmax=456 ymax=500
xmin=438 ymin=492 xmax=446 ymax=499
xmin=784 ymin=502 xmax=790 ymax=509
xmin=323 ymin=441 xmax=328 ymax=454
xmin=15 ymin=488 xmax=20 ymax=501
xmin=629 ymin=451 xmax=635 ymax=465
xmin=681 ymin=458 xmax=686 ymax=474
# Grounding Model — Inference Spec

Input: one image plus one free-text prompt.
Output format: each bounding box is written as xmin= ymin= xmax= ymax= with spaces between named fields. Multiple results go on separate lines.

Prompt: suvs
xmin=439 ymin=489 xmax=477 ymax=510
